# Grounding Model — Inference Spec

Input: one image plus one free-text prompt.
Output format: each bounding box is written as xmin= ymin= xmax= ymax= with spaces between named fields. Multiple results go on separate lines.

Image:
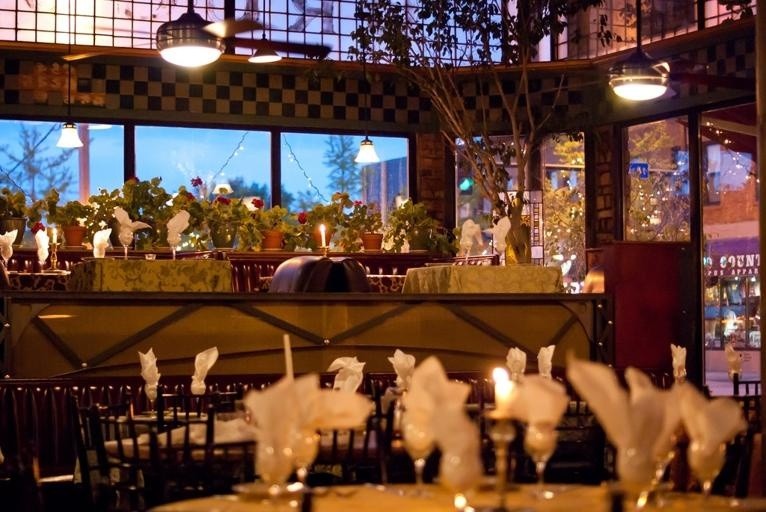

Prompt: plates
xmin=230 ymin=484 xmax=308 ymax=501
xmin=432 ymin=474 xmax=497 ymax=489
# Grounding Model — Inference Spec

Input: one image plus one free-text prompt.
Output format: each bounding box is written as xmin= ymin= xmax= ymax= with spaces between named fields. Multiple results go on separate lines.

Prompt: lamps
xmin=605 ymin=75 xmax=668 ymax=101
xmin=155 ymin=38 xmax=226 ymax=68
xmin=350 ymin=0 xmax=380 ymax=164
xmin=56 ymin=0 xmax=85 ymax=149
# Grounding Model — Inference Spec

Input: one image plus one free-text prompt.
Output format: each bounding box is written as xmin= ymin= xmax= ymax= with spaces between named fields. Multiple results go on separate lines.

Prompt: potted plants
xmin=0 ymin=171 xmax=462 ymax=255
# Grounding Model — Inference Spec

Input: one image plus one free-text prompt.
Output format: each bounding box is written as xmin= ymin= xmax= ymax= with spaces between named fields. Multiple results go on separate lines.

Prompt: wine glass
xmin=459 ymin=236 xmax=471 ymax=266
xmin=167 ymin=229 xmax=182 ymax=259
xmin=145 ymin=382 xmax=160 ymax=419
xmin=253 ymin=432 xmax=319 ymax=512
xmin=399 ymin=411 xmax=440 ymax=503
xmin=522 ymin=419 xmax=557 ymax=503
xmin=686 ymin=438 xmax=729 ymax=512
xmin=37 ymin=247 xmax=47 ymax=272
xmin=494 ymin=238 xmax=508 ymax=264
xmin=0 ymin=245 xmax=14 ymax=270
xmin=118 ymin=228 xmax=133 ymax=261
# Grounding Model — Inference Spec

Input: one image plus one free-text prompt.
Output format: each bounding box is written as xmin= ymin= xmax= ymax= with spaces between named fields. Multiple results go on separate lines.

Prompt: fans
xmin=538 ymin=0 xmax=754 ymax=102
xmin=60 ymin=0 xmax=332 ymax=62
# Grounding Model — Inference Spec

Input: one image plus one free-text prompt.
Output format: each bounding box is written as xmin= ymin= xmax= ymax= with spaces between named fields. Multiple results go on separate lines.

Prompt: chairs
xmin=0 ymin=374 xmax=763 ymax=511
xmin=0 ymin=251 xmax=609 ymax=294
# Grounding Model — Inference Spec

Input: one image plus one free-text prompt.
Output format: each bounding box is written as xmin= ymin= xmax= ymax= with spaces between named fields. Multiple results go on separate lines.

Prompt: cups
xmin=610 ymin=433 xmax=670 ymax=508
xmin=190 ymin=376 xmax=207 ymax=396
xmin=510 ymin=367 xmax=686 ymax=384
